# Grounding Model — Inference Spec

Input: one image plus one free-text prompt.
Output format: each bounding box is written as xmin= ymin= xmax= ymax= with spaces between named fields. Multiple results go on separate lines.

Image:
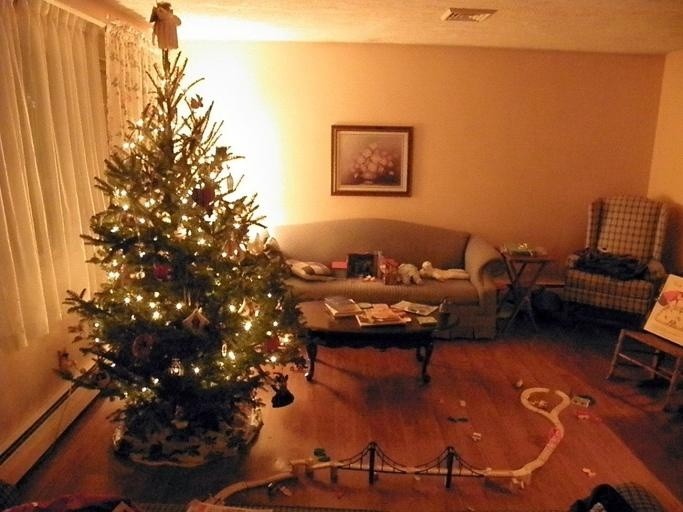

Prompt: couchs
xmin=244 ymin=218 xmax=504 ymax=343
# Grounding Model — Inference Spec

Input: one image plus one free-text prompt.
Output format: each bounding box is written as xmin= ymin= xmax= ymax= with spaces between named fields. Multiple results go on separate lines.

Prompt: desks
xmin=502 ymin=242 xmax=556 ymax=326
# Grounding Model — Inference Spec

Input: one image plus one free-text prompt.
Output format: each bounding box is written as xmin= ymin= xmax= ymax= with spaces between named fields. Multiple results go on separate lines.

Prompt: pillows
xmin=279 ymin=259 xmax=338 ymax=282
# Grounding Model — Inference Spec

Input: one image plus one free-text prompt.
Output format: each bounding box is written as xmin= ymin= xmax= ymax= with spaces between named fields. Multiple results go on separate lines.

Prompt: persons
xmin=657 ymin=291 xmax=683 ymax=307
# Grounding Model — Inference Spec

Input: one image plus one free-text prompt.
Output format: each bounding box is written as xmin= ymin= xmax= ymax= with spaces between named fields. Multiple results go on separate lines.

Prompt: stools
xmin=535 ymin=277 xmax=565 ymax=318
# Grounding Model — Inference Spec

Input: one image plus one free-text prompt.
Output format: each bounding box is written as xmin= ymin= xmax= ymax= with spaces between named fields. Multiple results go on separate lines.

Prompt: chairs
xmin=563 ymin=191 xmax=676 ymax=316
xmin=606 ymin=271 xmax=683 ymax=413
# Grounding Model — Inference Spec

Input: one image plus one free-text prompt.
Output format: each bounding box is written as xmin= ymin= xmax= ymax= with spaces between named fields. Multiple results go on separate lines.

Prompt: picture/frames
xmin=330 ymin=126 xmax=412 ymax=194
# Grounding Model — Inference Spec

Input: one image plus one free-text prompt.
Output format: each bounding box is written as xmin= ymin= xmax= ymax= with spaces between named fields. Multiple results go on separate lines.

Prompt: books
xmin=324 ymin=294 xmax=438 ymax=328
